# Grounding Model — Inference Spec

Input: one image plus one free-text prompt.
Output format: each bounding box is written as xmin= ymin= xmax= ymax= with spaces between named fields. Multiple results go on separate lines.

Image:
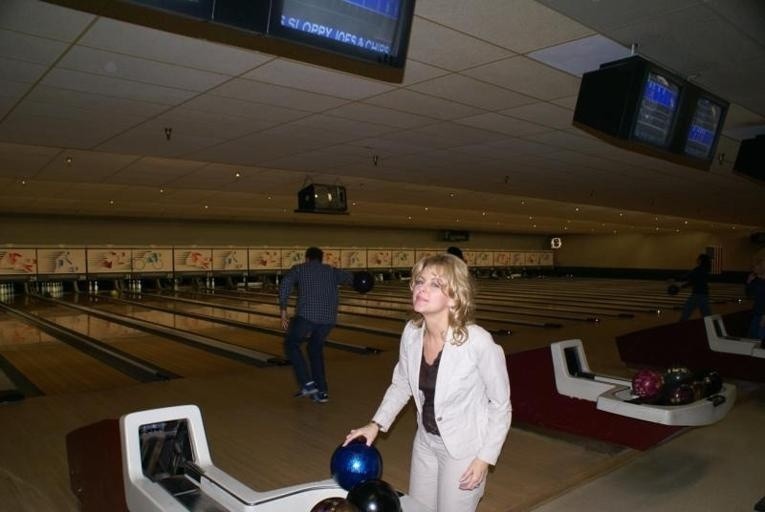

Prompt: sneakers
xmin=294 ymin=382 xmax=329 ymax=403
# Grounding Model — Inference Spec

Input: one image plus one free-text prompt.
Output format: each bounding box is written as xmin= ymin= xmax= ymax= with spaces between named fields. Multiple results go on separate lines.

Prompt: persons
xmin=743 ymin=248 xmax=765 ymax=349
xmin=277 ymin=247 xmax=353 ymax=404
xmin=342 ymin=254 xmax=514 ymax=510
xmin=675 ymin=252 xmax=714 ymax=323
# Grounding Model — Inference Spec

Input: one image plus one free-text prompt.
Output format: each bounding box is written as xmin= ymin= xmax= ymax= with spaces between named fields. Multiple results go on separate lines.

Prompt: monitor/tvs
xmin=268 ymin=1 xmax=415 ymax=68
xmin=574 ymin=57 xmax=683 ymax=169
xmin=668 ymin=78 xmax=731 ymax=175
xmin=731 ymin=130 xmax=765 ymax=183
xmin=298 ymin=182 xmax=346 ymax=212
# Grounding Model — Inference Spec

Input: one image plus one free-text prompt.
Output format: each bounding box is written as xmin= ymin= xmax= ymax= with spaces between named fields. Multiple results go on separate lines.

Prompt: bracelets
xmin=373 ymin=420 xmax=382 ymax=428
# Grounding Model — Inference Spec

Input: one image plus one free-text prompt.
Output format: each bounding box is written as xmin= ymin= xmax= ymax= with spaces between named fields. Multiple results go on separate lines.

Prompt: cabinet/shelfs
xmin=506 ymin=314 xmax=761 ymax=451
xmin=66 ymin=404 xmax=433 ymax=512
